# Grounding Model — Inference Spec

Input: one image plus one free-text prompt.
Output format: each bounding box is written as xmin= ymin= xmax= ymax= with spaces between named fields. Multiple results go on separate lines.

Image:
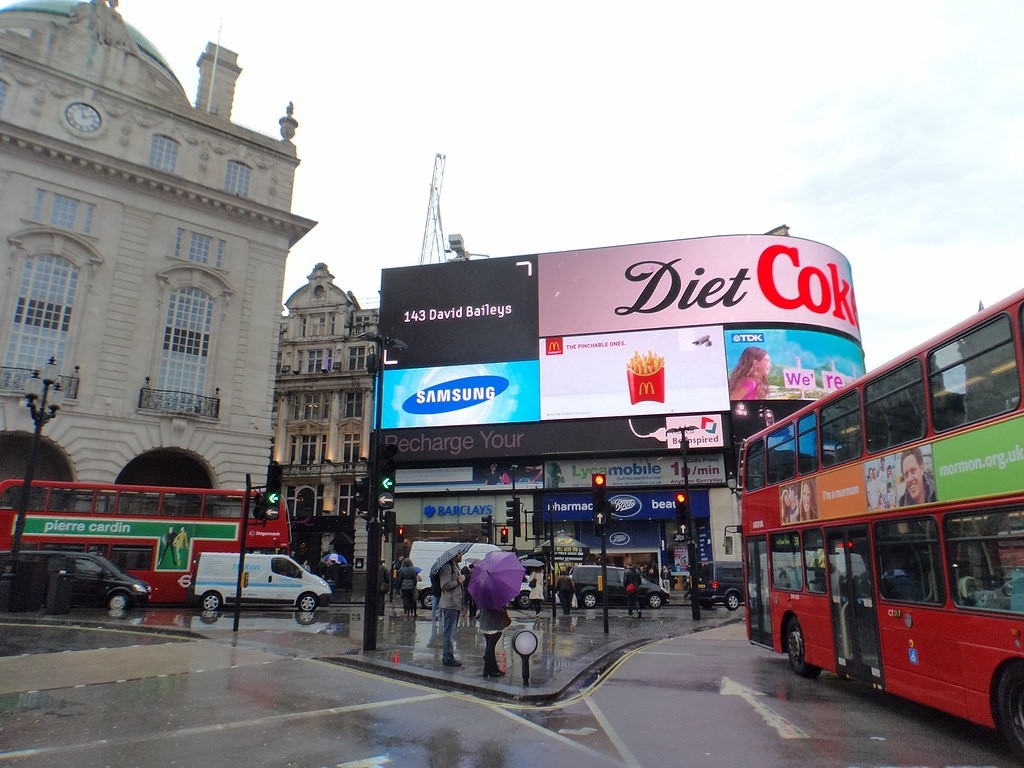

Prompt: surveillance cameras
xmin=692 ymin=335 xmax=710 ymax=346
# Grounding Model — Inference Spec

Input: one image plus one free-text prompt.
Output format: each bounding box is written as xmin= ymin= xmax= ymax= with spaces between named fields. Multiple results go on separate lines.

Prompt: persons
xmin=622 ymin=564 xmax=642 ymax=619
xmin=485 ymin=463 xmax=502 ymax=486
xmin=866 ymin=457 xmax=896 ymax=510
xmin=779 ymin=479 xmax=817 ymax=523
xmin=379 ymin=557 xmax=423 ymax=617
xmin=430 ymin=553 xmax=477 ymax=667
xmin=729 ymin=347 xmax=771 ymax=400
xmin=499 ymin=471 xmax=510 ymax=484
xmin=639 ymin=565 xmax=659 ymax=586
xmin=477 ymin=607 xmax=511 ymax=678
xmin=524 ymin=465 xmax=542 ymax=484
xmin=302 ymin=560 xmax=310 ymax=571
xmin=554 ymin=571 xmax=574 ymax=615
xmin=660 ymin=564 xmax=671 ymax=592
xmin=318 ymin=559 xmax=337 ymax=580
xmin=898 ymin=447 xmax=936 ymax=507
xmin=524 ymin=566 xmax=545 ymax=616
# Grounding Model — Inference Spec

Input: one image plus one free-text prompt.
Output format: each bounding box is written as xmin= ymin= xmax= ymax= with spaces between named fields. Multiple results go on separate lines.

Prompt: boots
xmin=483 ymin=656 xmax=505 ymax=677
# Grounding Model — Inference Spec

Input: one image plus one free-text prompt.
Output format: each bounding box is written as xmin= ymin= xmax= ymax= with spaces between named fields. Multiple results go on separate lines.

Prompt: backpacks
xmin=431 ymin=562 xmax=453 ymax=598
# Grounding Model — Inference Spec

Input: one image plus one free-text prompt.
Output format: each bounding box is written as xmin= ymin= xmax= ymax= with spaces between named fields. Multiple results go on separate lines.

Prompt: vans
xmin=194 ymin=552 xmax=332 ymax=612
xmin=0 ymin=551 xmax=151 ymax=611
xmin=698 ymin=561 xmax=746 ymax=611
xmin=409 ymin=541 xmax=535 ymax=610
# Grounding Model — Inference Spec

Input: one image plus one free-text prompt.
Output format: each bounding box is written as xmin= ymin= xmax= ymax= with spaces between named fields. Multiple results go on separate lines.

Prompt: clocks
xmin=65 ymin=101 xmax=103 ymax=133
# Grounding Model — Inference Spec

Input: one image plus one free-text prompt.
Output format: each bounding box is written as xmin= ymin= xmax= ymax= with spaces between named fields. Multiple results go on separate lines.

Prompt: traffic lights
xmin=396 ymin=528 xmax=404 ymax=543
xmin=374 ymin=443 xmax=397 ymax=510
xmin=592 ymin=473 xmax=608 ymax=526
xmin=385 ymin=512 xmax=396 ymax=533
xmin=501 ymin=529 xmax=508 ymax=543
xmin=481 ymin=515 xmax=492 ymax=544
xmin=263 ymin=465 xmax=283 ymax=520
xmin=355 ymin=477 xmax=369 ymax=511
xmin=674 ymin=491 xmax=690 ymax=535
xmin=506 ymin=497 xmax=521 ymax=537
xmin=253 ymin=492 xmax=265 ymax=521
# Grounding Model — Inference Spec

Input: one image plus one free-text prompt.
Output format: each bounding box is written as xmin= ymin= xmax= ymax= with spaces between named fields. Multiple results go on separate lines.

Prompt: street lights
xmin=355 ymin=332 xmax=409 ymax=653
xmin=667 ymin=426 xmax=701 ymax=621
xmin=1 ymin=356 xmax=65 ymax=613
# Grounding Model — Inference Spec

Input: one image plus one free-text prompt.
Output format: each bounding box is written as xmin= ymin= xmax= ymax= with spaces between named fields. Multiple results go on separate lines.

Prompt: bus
xmin=0 ymin=479 xmax=292 ymax=603
xmin=723 ymin=286 xmax=1024 ymax=766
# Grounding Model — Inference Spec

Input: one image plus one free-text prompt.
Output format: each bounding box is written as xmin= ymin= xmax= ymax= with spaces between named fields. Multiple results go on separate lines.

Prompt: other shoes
xmin=638 ymin=611 xmax=642 ymax=618
xmin=629 ymin=613 xmax=633 ymax=615
xmin=443 ymin=660 xmax=462 ymax=666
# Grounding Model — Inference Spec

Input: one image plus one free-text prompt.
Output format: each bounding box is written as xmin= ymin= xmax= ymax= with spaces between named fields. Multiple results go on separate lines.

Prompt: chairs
xmin=957 ymin=574 xmax=981 ymax=606
xmin=761 ymin=390 xmax=1005 ymax=483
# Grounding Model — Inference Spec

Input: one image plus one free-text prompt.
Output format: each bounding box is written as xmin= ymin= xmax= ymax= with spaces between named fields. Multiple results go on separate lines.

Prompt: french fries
xmin=626 ymin=350 xmax=664 ymax=374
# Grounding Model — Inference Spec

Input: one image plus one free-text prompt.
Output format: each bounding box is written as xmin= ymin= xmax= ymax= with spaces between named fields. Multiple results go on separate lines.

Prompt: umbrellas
xmin=323 ymin=553 xmax=347 ymax=565
xmin=520 ymin=558 xmax=545 ymax=567
xmin=429 ymin=542 xmax=475 ymax=575
xmin=467 ymin=551 xmax=526 ymax=609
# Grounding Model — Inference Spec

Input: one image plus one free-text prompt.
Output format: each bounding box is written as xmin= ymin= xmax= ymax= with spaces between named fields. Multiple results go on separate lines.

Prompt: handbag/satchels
xmin=500 ymin=607 xmax=511 ymax=627
xmin=626 ymin=584 xmax=635 ymax=592
xmin=555 ymin=591 xmax=560 ymax=604
xmin=380 ymin=582 xmax=389 ymax=593
xmin=529 ymin=579 xmax=536 ymax=588
xmin=412 ymin=589 xmax=419 ymax=601
xmin=572 ymin=593 xmax=578 ymax=608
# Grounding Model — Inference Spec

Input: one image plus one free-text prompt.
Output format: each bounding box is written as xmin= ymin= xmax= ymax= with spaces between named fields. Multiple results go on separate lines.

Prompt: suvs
xmin=572 ymin=565 xmax=670 ymax=609
